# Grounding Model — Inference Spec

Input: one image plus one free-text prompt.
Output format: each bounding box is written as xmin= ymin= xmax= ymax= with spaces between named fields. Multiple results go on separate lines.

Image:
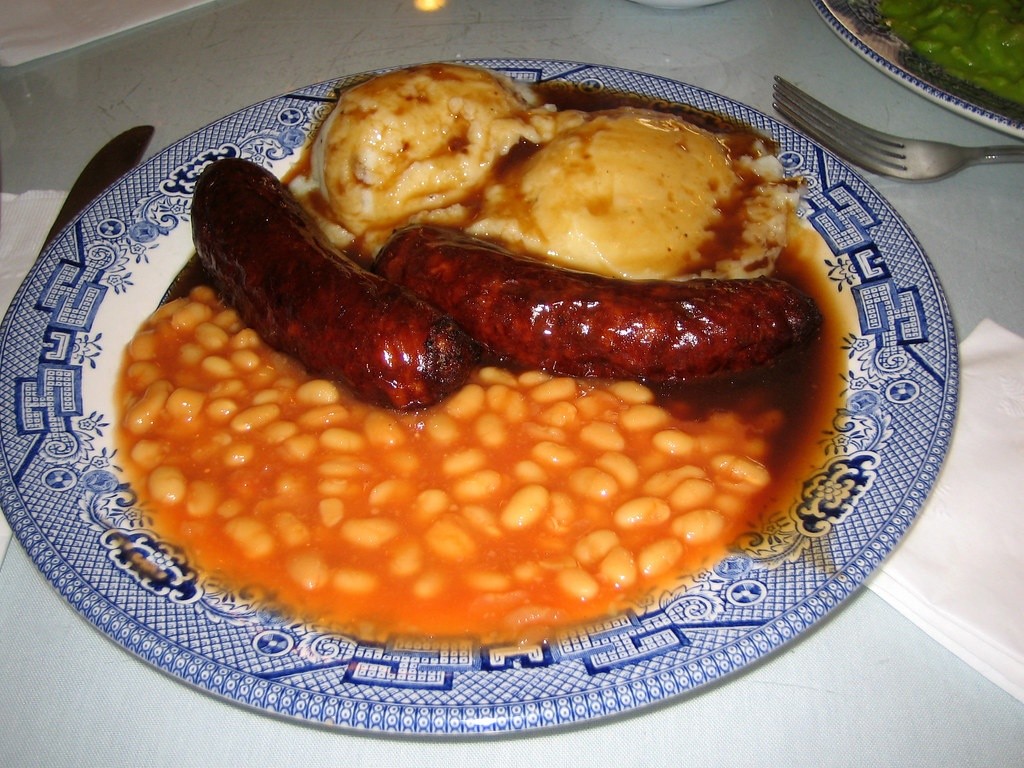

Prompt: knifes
xmin=39 ymin=125 xmax=156 ymax=255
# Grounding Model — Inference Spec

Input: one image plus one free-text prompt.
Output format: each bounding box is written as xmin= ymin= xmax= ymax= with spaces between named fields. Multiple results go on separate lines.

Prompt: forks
xmin=771 ymin=74 xmax=1023 ymax=184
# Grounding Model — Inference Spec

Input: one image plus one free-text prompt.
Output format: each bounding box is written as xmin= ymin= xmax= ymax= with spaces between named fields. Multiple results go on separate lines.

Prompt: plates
xmin=809 ymin=0 xmax=1024 ymax=138
xmin=1 ymin=57 xmax=960 ymax=737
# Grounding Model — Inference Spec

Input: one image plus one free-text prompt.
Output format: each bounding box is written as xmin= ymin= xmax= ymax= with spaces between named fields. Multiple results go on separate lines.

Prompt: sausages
xmin=190 ymin=158 xmax=488 ymax=411
xmin=368 ymin=222 xmax=823 ymax=386
